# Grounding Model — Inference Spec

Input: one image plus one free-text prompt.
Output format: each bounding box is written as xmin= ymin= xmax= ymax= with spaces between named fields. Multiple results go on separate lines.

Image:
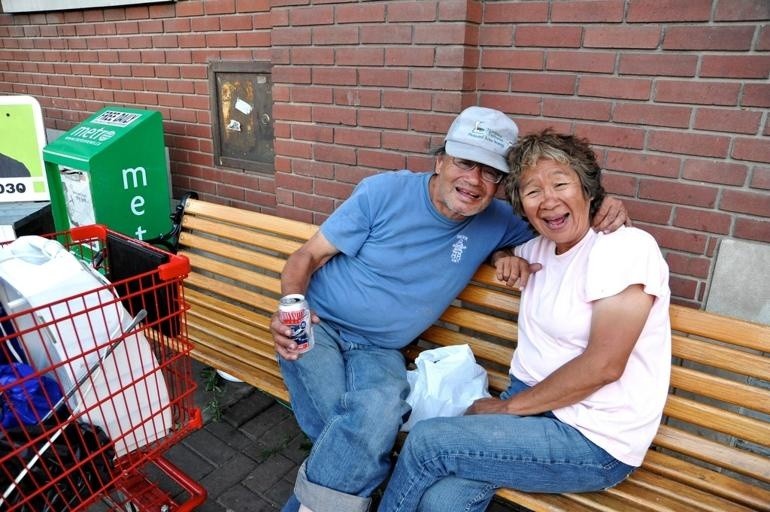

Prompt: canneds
xmin=278 ymin=293 xmax=314 ymax=354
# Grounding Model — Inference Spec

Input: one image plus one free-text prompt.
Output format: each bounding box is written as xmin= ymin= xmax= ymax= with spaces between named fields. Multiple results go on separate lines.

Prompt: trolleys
xmin=0 ymin=223 xmax=207 ymax=511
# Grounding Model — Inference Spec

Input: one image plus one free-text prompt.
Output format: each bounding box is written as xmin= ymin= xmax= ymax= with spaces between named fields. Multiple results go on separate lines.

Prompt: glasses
xmin=452 ymin=156 xmax=505 ymax=184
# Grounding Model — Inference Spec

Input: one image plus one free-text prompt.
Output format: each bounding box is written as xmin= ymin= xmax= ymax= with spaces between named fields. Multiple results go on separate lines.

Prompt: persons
xmin=376 ymin=128 xmax=674 ymax=512
xmin=267 ymin=104 xmax=634 ymax=512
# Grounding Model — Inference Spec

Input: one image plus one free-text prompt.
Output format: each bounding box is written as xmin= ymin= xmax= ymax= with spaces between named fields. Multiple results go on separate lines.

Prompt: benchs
xmin=89 ymin=192 xmax=770 ymax=512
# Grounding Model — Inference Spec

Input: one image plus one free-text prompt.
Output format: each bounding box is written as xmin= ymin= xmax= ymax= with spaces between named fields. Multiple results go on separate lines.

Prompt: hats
xmin=443 ymin=105 xmax=520 ymax=174
